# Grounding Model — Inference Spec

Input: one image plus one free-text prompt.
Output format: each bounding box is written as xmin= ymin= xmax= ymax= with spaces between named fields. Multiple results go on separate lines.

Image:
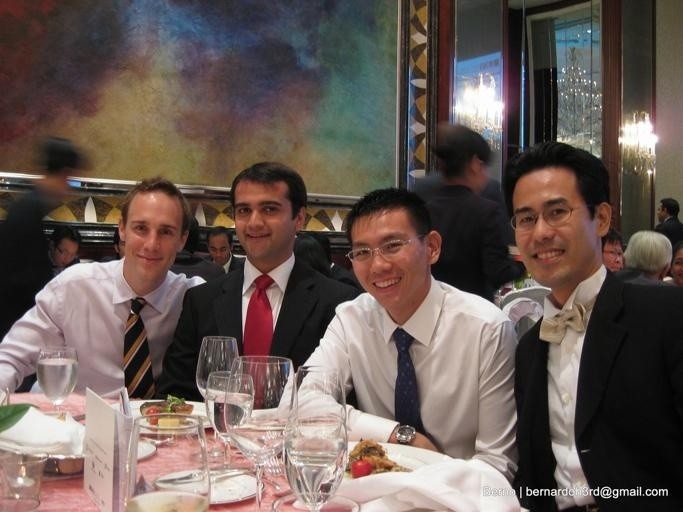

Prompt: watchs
xmin=393 ymin=423 xmax=418 ymax=443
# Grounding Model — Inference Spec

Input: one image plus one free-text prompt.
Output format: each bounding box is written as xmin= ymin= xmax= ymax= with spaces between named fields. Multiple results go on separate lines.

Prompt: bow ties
xmin=539 ymin=296 xmax=597 ymax=346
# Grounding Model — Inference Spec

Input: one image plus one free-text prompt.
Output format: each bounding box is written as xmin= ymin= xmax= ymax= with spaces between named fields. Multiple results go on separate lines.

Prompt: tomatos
xmin=145 ymin=408 xmax=161 ymax=426
xmin=350 ymin=455 xmax=371 ymax=480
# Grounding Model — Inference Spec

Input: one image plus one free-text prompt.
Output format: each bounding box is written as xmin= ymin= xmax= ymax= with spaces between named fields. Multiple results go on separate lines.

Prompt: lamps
xmin=616 ymin=107 xmax=660 ymax=180
xmin=450 ymin=71 xmax=505 ymax=153
xmin=555 ymin=42 xmax=602 ymax=153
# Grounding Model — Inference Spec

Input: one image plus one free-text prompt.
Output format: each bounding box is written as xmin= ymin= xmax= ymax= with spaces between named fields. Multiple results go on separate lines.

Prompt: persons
xmin=151 ymin=160 xmax=359 ymax=409
xmin=504 ymin=141 xmax=683 ymax=511
xmin=1 ymin=137 xmax=97 ymax=394
xmin=0 ymin=175 xmax=208 ymax=401
xmin=279 ymin=186 xmax=520 ymax=487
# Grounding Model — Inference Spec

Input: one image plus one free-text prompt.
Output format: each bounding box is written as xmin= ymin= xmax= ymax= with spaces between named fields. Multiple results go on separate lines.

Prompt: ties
xmin=123 ymin=298 xmax=158 ymax=400
xmin=243 ymin=275 xmax=277 ymax=411
xmin=392 ymin=328 xmax=426 ymax=436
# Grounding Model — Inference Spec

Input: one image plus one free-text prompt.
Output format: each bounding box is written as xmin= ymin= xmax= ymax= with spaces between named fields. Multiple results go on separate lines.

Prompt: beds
xmin=0 ymin=390 xmax=526 ymax=512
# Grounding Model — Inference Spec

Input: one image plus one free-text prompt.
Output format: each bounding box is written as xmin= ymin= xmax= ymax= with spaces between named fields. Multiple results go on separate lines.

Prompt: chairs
xmin=497 ymin=285 xmax=553 ymax=342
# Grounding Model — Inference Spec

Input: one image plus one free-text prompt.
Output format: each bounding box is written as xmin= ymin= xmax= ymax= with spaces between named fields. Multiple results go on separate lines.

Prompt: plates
xmin=342 ymin=454 xmax=427 ymax=483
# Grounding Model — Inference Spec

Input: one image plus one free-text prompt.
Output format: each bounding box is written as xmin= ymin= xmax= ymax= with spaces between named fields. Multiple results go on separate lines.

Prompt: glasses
xmin=509 ymin=199 xmax=589 ymax=233
xmin=601 ymin=247 xmax=624 ymax=261
xmin=54 ymin=243 xmax=79 ymax=259
xmin=344 ymin=232 xmax=428 ymax=263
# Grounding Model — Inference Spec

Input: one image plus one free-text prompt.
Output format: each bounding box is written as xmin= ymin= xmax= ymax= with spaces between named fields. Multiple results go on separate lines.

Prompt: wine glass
xmin=0 ymin=334 xmax=360 ymax=512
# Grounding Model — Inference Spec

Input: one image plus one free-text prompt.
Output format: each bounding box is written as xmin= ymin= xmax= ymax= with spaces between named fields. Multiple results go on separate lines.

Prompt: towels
xmin=1 ymin=404 xmax=76 ymax=458
xmin=332 ymin=435 xmax=522 ymax=512
